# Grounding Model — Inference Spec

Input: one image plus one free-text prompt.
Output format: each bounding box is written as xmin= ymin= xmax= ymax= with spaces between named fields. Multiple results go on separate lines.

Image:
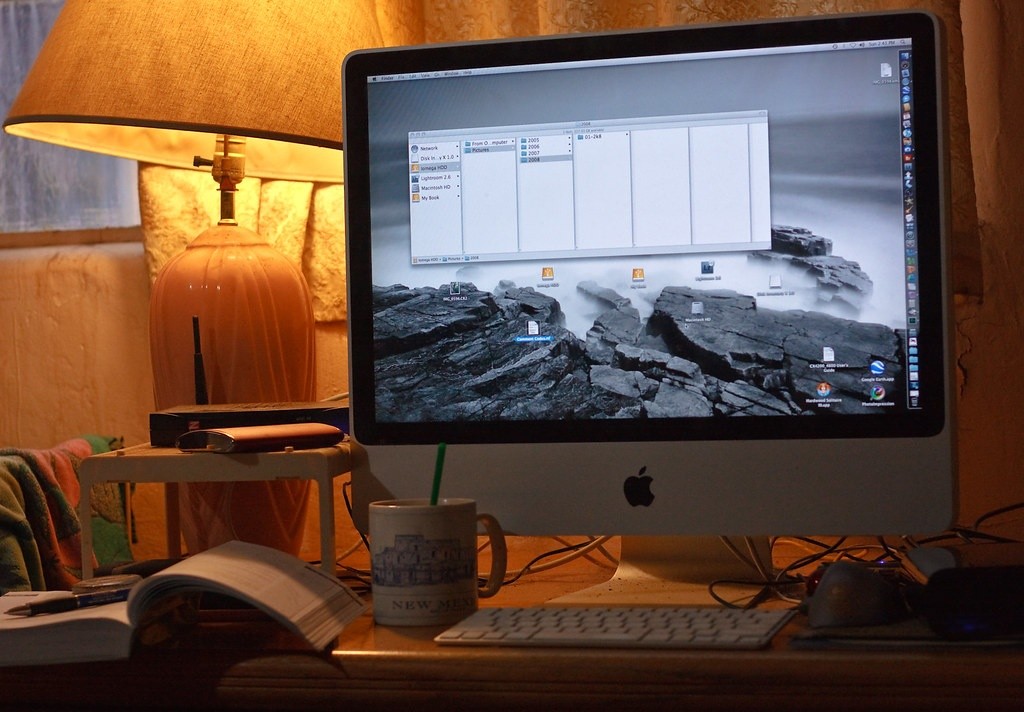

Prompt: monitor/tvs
xmin=342 ymin=6 xmax=960 ymax=609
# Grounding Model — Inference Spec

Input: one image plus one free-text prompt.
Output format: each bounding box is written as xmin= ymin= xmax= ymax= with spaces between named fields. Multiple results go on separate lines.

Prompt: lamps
xmin=2 ymin=0 xmax=385 ymax=557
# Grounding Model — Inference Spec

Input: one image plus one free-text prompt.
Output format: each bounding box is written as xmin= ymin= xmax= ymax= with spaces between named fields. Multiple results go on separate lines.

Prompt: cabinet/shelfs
xmin=78 ymin=443 xmax=351 ymax=580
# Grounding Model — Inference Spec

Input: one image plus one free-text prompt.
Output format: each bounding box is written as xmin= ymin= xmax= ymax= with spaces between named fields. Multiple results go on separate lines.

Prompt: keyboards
xmin=435 ymin=606 xmax=801 ymax=650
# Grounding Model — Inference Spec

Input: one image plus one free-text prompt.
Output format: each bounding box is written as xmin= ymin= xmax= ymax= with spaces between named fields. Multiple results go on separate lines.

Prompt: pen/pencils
xmin=3 ymin=586 xmax=132 ymax=618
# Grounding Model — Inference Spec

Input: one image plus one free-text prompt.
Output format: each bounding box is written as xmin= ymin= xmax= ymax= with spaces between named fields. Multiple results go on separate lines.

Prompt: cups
xmin=371 ymin=498 xmax=507 ymax=623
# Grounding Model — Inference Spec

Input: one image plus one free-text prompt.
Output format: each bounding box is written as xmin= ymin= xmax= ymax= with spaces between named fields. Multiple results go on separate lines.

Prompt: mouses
xmin=807 ymin=562 xmax=903 ymax=627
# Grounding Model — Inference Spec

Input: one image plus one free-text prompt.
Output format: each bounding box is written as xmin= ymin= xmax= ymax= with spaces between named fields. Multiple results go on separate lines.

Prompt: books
xmin=0 ymin=541 xmax=368 ymax=668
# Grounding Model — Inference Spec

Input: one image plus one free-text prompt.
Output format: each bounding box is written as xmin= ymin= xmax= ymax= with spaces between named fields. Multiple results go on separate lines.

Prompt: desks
xmin=0 ymin=530 xmax=1024 ymax=712
